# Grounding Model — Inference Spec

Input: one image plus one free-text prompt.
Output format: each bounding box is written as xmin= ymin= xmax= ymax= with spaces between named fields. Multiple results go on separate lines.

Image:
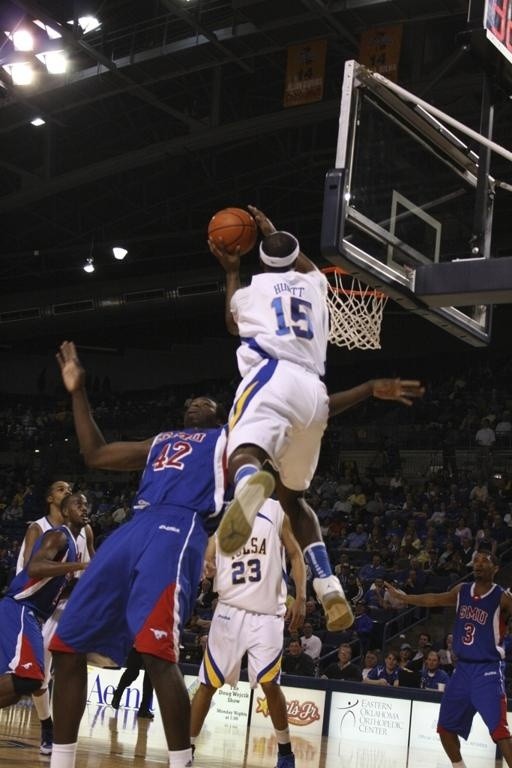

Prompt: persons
xmin=14 ymin=482 xmax=97 ymax=756
xmin=111 ymin=609 xmax=212 ymax=718
xmin=50 ymin=337 xmax=426 ymax=768
xmin=178 ymin=576 xmax=216 ymax=665
xmin=187 ymin=495 xmax=307 ymax=768
xmin=285 ymin=381 xmax=512 ymax=704
xmin=0 ymin=491 xmax=92 ymax=706
xmin=206 ymin=203 xmax=356 ymax=636
xmin=0 ymin=374 xmax=242 ymax=606
xmin=382 ymin=548 xmax=512 ymax=768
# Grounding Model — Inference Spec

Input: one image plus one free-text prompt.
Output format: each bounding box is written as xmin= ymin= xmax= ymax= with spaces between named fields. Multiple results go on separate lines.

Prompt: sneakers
xmin=273 ymin=751 xmax=294 ymax=768
xmin=112 ymin=689 xmax=122 ymax=709
xmin=136 ymin=708 xmax=155 ymax=718
xmin=312 ymin=574 xmax=354 ymax=632
xmin=218 ymin=470 xmax=276 ymax=553
xmin=40 ymin=729 xmax=52 ymax=754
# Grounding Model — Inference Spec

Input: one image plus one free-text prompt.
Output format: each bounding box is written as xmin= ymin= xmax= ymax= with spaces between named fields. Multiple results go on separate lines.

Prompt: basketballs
xmin=209 ymin=208 xmax=257 ymax=255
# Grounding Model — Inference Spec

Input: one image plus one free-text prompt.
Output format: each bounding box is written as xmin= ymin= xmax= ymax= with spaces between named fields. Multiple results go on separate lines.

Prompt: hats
xmin=400 ymin=643 xmax=412 ymax=651
